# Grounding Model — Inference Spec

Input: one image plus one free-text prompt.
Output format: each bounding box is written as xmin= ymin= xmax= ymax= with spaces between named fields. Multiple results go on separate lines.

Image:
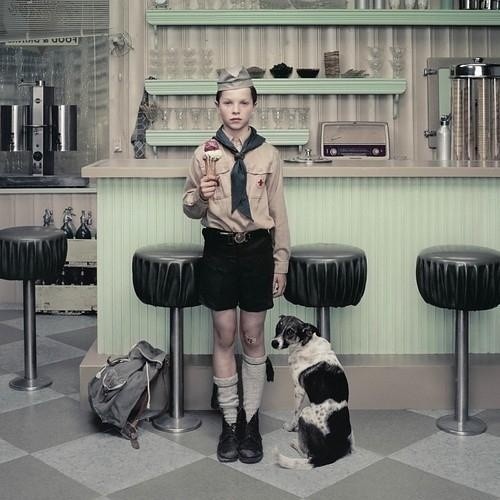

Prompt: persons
xmin=180 ymin=63 xmax=291 ymax=463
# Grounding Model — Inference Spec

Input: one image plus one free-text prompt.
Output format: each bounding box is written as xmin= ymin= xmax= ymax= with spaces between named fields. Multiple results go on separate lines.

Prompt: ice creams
xmin=202 ymin=139 xmax=221 ymax=183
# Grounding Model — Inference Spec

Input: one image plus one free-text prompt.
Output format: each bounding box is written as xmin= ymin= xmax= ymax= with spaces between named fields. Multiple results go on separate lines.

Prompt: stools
xmin=2 ymin=226 xmax=68 ymax=392
xmin=416 ymin=242 xmax=500 ymax=435
xmin=285 ymin=244 xmax=368 ymax=343
xmin=132 ymin=243 xmax=203 ymax=435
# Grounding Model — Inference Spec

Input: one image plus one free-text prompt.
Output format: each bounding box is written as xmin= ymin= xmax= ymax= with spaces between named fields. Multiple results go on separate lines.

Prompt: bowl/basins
xmin=270 ymin=67 xmax=293 ymax=78
xmin=296 ymin=68 xmax=320 ymax=79
xmin=247 ymin=71 xmax=266 ymax=78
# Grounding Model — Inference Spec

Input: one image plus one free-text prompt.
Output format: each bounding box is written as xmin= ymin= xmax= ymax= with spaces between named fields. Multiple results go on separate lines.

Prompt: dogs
xmin=271 ymin=315 xmax=356 ymax=470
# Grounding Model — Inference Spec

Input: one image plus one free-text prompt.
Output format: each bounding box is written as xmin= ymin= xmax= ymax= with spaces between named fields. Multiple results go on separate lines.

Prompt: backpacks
xmin=86 ymin=341 xmax=172 ymax=441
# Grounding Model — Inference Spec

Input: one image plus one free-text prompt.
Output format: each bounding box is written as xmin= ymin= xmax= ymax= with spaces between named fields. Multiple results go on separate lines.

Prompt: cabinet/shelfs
xmin=145 ymin=7 xmax=500 ymax=155
xmin=35 ymin=236 xmax=97 ymax=315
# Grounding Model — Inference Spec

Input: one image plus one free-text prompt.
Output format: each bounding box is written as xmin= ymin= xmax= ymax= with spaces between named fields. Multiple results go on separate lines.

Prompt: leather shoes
xmin=240 ymin=407 xmax=264 ymax=464
xmin=216 ymin=405 xmax=241 ymax=463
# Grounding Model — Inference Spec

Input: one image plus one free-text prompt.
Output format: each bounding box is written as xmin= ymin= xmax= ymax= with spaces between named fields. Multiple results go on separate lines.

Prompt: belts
xmin=218 ymin=228 xmax=269 ymax=245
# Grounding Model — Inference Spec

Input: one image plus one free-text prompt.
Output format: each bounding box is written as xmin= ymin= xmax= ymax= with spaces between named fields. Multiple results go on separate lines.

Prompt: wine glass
xmin=157 ymin=107 xmax=310 ymax=130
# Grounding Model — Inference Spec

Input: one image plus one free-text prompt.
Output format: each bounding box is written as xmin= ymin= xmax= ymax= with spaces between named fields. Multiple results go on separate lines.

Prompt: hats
xmin=216 ymin=64 xmax=255 ymax=92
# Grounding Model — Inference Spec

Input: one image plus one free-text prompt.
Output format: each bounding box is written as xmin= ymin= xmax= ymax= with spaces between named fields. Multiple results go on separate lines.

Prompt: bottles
xmin=435 ymin=121 xmax=451 ymax=160
